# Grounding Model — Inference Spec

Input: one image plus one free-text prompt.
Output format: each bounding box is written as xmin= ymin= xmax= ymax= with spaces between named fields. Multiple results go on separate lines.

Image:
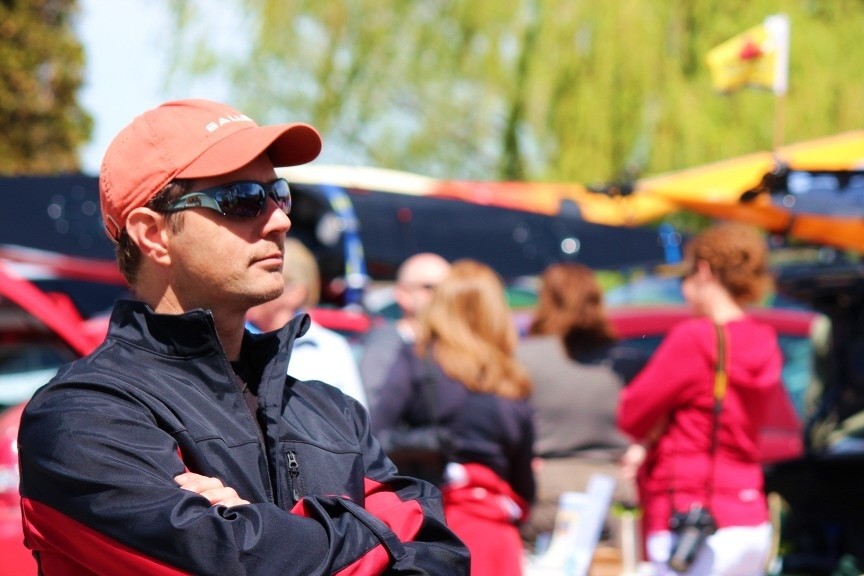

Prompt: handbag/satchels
xmin=440 ymin=462 xmax=531 ymax=576
xmin=667 ymin=506 xmax=717 ymax=575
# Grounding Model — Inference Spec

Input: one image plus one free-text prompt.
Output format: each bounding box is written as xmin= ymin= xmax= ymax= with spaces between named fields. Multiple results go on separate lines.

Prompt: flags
xmin=705 ymin=22 xmax=777 ymax=95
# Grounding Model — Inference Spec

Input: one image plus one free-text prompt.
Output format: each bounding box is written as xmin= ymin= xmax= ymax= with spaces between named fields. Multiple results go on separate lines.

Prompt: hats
xmin=99 ymin=98 xmax=323 ymax=245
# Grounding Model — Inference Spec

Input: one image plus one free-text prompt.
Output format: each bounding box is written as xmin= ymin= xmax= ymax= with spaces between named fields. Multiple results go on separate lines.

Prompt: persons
xmin=17 ymin=98 xmax=471 ymax=576
xmin=244 ymin=220 xmax=864 ymax=576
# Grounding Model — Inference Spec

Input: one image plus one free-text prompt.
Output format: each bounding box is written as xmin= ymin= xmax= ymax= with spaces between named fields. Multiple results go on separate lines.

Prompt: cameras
xmin=668 ymin=506 xmax=717 ymax=573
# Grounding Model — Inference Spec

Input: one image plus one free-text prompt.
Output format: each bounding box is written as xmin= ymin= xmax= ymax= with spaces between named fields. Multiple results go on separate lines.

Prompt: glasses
xmin=153 ymin=178 xmax=292 ymax=219
